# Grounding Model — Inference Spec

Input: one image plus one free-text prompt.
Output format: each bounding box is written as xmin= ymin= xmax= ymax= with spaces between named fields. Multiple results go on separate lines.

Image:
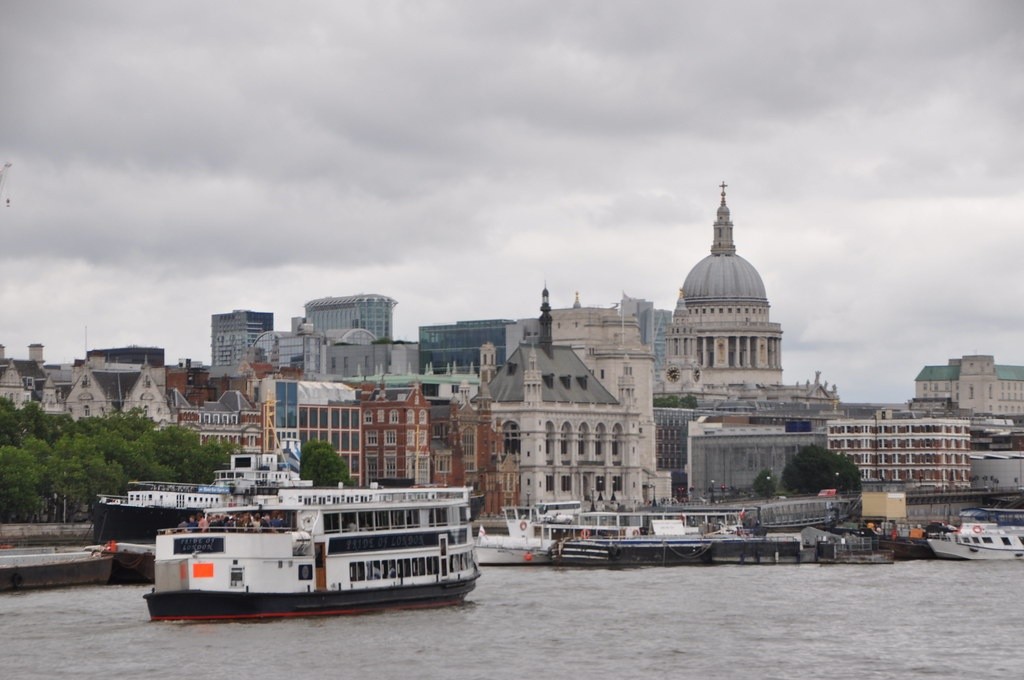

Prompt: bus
xmin=532 ymin=501 xmax=582 ymax=520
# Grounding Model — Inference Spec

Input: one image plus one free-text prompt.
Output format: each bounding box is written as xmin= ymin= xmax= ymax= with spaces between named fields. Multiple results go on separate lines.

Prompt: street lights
xmin=710 ymin=480 xmax=715 ymax=504
xmin=596 ymin=479 xmax=604 ymax=502
xmin=526 ymin=491 xmax=532 ymax=513
xmin=590 ymin=486 xmax=597 ymax=512
xmin=610 ymin=480 xmax=616 ymax=501
xmin=651 ymin=483 xmax=658 ymax=508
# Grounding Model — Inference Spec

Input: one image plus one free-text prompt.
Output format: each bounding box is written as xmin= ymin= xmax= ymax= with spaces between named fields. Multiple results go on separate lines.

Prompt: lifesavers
xmin=633 ymin=529 xmax=639 ymax=536
xmin=301 ymin=515 xmax=315 ymax=532
xmin=973 ymin=525 xmax=982 ymax=533
xmin=580 ymin=530 xmax=590 ymax=538
xmin=520 ymin=522 xmax=527 ymax=532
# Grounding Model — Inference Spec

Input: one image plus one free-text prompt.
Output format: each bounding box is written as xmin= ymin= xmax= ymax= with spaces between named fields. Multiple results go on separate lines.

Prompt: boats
xmin=82 ymin=390 xmax=861 ymax=621
xmin=927 ymin=521 xmax=1024 ymax=561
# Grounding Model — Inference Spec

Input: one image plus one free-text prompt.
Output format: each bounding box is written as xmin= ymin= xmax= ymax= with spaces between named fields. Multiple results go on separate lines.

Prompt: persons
xmin=185 ymin=512 xmax=289 ymax=533
